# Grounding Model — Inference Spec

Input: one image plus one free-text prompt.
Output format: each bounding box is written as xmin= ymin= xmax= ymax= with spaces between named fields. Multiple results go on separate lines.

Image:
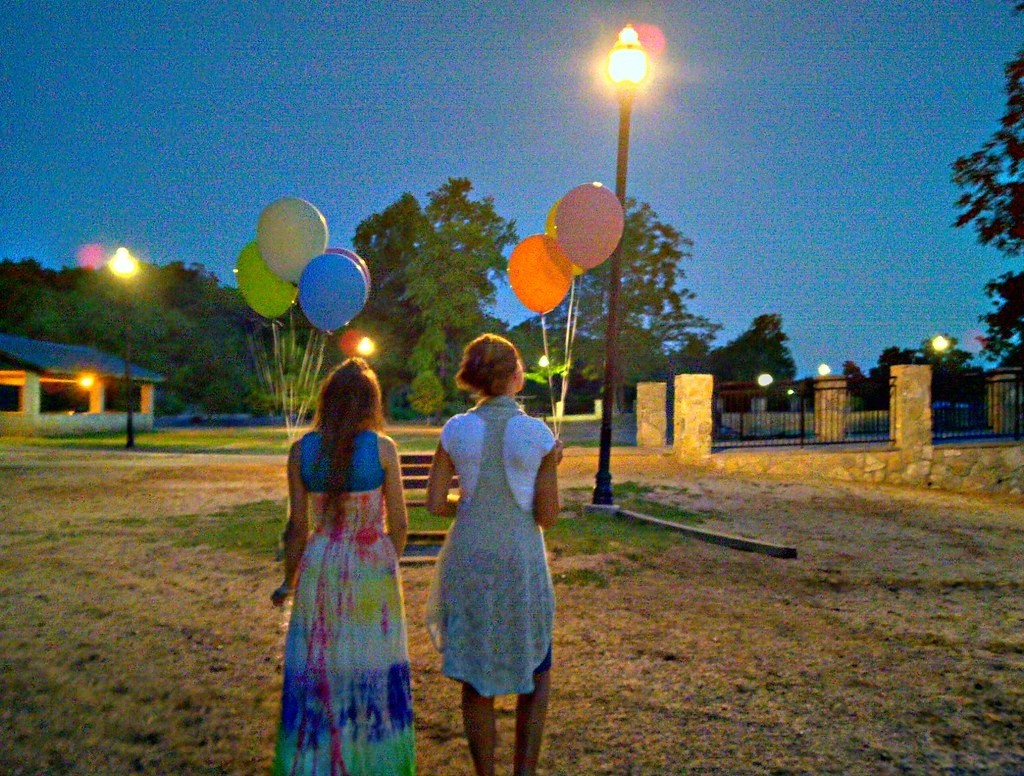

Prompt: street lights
xmin=107 ymin=245 xmax=142 ymax=451
xmin=589 ymin=24 xmax=652 ymax=516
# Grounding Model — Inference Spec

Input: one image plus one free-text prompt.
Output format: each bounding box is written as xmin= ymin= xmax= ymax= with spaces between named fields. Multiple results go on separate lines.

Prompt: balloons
xmin=507 ymin=233 xmax=572 ymax=314
xmin=545 ymin=183 xmax=624 ymax=278
xmin=299 ymin=247 xmax=370 ymax=332
xmin=235 ymin=240 xmax=299 ymax=319
xmin=257 ymin=196 xmax=329 ymax=286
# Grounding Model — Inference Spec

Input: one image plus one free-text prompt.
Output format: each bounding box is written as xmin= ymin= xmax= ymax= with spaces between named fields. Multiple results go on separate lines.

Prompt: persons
xmin=270 ymin=356 xmax=418 ymax=776
xmin=424 ymin=334 xmax=564 ymax=776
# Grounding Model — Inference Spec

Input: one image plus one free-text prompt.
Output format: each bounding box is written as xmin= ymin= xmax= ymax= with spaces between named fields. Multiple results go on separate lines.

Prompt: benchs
xmin=399 ymin=453 xmax=459 ymax=541
